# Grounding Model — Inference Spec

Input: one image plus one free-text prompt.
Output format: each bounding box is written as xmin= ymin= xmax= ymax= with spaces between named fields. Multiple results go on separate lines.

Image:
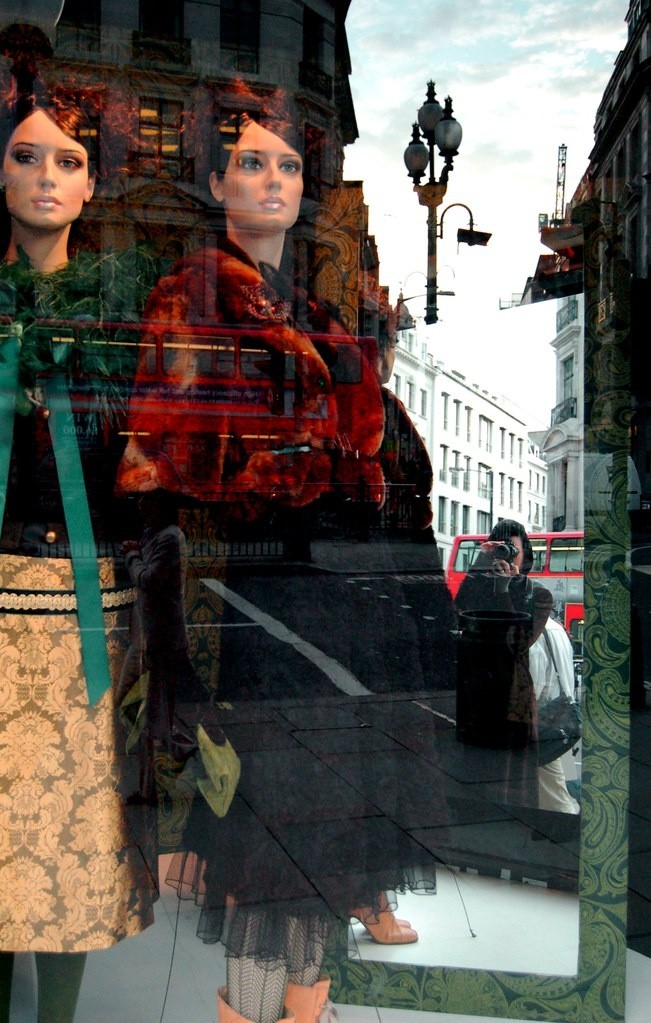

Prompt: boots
xmin=282 ymin=972 xmax=330 ymax=1023
xmin=216 ymin=983 xmax=295 ymax=1023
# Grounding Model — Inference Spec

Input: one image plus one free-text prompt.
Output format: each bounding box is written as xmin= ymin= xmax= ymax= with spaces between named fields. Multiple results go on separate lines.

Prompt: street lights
xmin=404 ymin=79 xmax=492 ymax=326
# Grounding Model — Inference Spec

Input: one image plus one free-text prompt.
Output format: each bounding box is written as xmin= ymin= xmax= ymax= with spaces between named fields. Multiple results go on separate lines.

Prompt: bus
xmin=444 ymin=532 xmax=583 ymax=679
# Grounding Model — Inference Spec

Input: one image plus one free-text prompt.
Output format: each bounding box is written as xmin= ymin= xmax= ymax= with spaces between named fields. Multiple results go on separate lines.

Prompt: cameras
xmin=493 ymin=539 xmax=519 ymax=571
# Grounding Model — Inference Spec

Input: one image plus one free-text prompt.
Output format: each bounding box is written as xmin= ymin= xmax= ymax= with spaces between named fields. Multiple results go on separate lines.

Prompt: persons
xmin=528 ymin=579 xmax=582 ymax=815
xmin=0 ymin=50 xmax=460 ymax=1023
xmin=452 ymin=519 xmax=553 ymax=808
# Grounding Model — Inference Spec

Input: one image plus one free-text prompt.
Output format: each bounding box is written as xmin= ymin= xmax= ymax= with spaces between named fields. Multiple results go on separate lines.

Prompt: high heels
xmin=346 ymin=890 xmax=418 ymax=944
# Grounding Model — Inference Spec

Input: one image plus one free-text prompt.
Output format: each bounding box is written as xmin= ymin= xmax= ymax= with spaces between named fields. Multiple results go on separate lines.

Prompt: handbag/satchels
xmin=535 ymin=694 xmax=581 ymax=768
